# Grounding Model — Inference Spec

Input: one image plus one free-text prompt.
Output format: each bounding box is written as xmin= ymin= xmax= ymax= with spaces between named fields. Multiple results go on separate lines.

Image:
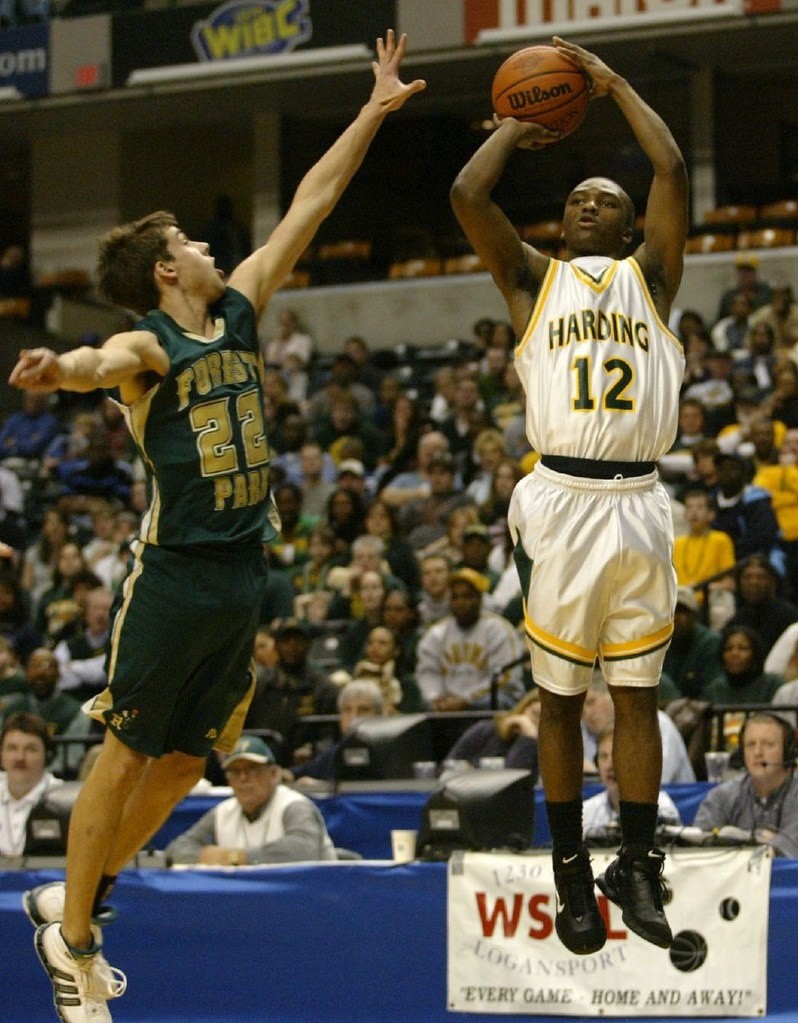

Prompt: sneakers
xmin=22 ymin=880 xmax=68 ymax=927
xmin=551 ymin=845 xmax=608 ymax=956
xmin=34 ymin=919 xmax=128 ymax=1023
xmin=595 ymin=845 xmax=673 ymax=949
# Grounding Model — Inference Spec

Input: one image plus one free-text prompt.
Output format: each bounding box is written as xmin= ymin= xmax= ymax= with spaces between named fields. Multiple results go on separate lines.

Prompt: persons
xmin=581 ymin=721 xmax=682 ymax=842
xmin=693 ymin=712 xmax=798 ymax=861
xmin=0 ymin=710 xmax=69 ymax=859
xmin=9 ymin=26 xmax=425 ymax=1023
xmin=165 ymin=735 xmax=338 ymax=867
xmin=0 ymin=254 xmax=798 ymax=793
xmin=450 ymin=36 xmax=691 ymax=957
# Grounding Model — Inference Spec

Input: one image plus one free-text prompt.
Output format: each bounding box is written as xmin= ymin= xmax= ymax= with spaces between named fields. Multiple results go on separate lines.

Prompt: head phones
xmin=737 ymin=712 xmax=798 ymax=768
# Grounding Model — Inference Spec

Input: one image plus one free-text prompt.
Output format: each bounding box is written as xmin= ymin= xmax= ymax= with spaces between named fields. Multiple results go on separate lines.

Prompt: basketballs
xmin=491 ymin=45 xmax=589 ymax=144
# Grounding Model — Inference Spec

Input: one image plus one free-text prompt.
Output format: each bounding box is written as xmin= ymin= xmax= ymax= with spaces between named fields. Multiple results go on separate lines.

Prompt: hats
xmin=463 ymin=524 xmax=492 ymax=543
xmin=220 ymin=735 xmax=276 ymax=769
xmin=449 ymin=566 xmax=485 ymax=592
xmin=337 ymin=458 xmax=364 ymax=476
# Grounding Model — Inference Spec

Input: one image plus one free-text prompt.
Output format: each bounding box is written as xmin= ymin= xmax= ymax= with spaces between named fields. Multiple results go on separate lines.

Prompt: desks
xmin=143 ymin=779 xmax=727 ymax=854
xmin=0 ymin=850 xmax=798 ymax=1023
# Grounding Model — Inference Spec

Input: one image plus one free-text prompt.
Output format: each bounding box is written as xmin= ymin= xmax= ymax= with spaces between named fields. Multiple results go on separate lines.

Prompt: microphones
xmin=760 ymin=760 xmax=798 ymax=769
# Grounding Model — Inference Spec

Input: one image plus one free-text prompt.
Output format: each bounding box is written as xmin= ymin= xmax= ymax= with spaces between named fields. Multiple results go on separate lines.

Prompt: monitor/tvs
xmin=423 ymin=768 xmax=537 ymax=851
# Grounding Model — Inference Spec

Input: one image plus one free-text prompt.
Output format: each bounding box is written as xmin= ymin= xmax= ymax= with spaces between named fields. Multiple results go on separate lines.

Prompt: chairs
xmin=276 ymin=199 xmax=798 ymax=289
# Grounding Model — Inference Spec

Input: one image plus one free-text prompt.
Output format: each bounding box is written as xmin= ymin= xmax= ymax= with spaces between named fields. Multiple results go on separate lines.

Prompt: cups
xmin=479 ymin=756 xmax=505 ymax=770
xmin=412 ymin=761 xmax=435 ymax=777
xmin=705 ymin=752 xmax=730 ymax=783
xmin=390 ymin=829 xmax=418 ymax=862
